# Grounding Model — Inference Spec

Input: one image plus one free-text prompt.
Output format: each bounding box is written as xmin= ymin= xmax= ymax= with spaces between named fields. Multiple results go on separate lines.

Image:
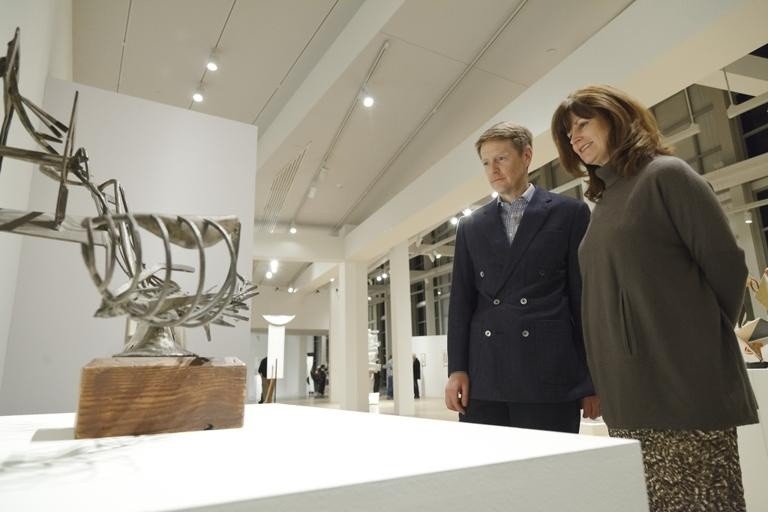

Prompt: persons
xmin=258 ymin=356 xmax=266 ymax=403
xmin=550 ymin=86 xmax=760 ymax=512
xmin=383 ymin=356 xmax=394 ymax=398
xmin=413 ymin=355 xmax=422 ymax=400
xmin=318 ymin=364 xmax=327 ymax=398
xmin=311 ymin=362 xmax=318 ymax=393
xmin=444 ymin=122 xmax=602 ymax=434
xmin=374 ymin=359 xmax=380 ymax=392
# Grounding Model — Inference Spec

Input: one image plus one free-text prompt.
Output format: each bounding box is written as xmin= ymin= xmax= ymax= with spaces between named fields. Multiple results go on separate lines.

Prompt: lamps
xmin=207 ymin=50 xmax=222 ymax=71
xmin=289 ymin=41 xmax=391 ymax=234
xmin=192 ymin=87 xmax=206 ymax=102
xmin=433 ymin=250 xmax=442 ymax=258
